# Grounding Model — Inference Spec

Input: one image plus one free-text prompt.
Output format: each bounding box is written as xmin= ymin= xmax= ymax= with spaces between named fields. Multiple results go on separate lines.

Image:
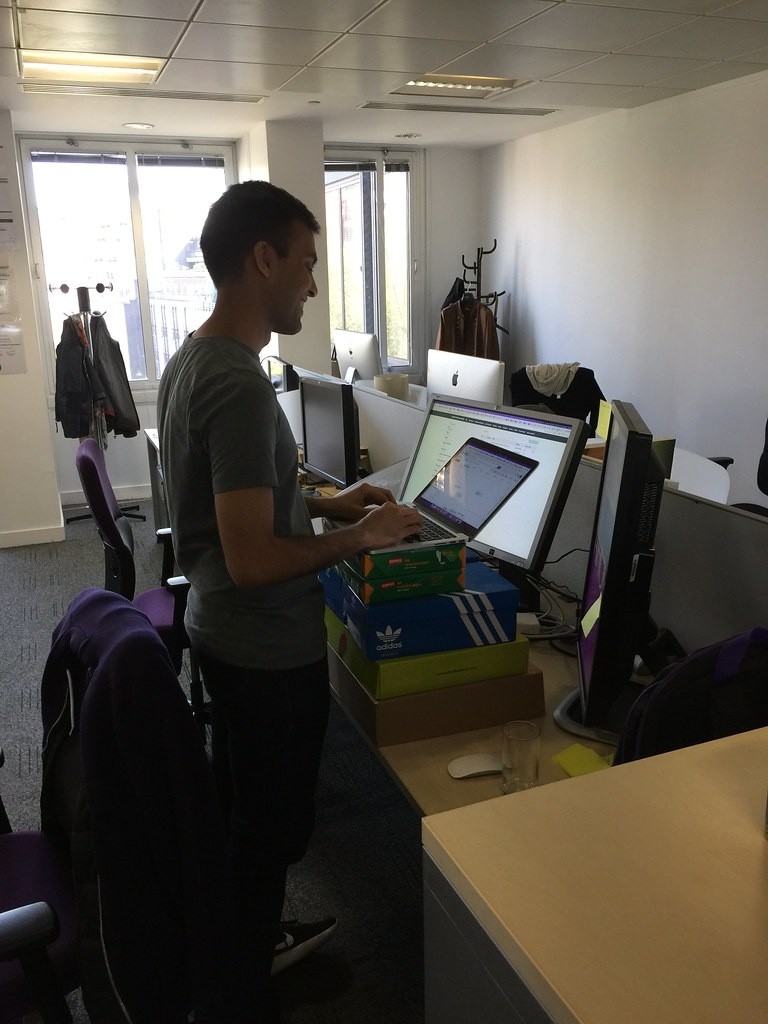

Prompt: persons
xmin=160 ymin=183 xmax=424 ymax=975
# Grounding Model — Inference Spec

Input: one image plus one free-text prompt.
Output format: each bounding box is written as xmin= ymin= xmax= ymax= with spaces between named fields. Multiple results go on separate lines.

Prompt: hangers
xmin=460 ymin=274 xmax=475 ymax=309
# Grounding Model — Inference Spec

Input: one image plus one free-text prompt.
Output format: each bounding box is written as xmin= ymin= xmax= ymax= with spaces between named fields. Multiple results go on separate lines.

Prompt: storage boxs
xmin=317 ymin=548 xmax=478 ymax=620
xmin=341 ymin=563 xmax=519 ymax=661
xmin=330 ymin=559 xmax=467 ymax=604
xmin=326 ymin=641 xmax=546 ymax=745
xmin=321 ymin=501 xmax=468 ymax=578
xmin=324 ymin=604 xmax=531 ymax=699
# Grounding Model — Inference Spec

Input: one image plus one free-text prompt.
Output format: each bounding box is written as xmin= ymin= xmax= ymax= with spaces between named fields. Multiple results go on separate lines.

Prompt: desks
xmin=144 ymin=427 xmax=621 ymax=823
xmin=421 ymin=726 xmax=768 ymax=1024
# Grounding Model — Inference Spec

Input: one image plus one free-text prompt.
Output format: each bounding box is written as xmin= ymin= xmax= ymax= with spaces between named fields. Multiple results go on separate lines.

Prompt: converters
xmin=516 ymin=613 xmax=540 ymax=634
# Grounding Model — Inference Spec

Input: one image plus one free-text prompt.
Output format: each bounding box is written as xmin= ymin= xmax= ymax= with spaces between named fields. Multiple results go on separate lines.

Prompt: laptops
xmin=322 ymin=438 xmax=539 ymax=555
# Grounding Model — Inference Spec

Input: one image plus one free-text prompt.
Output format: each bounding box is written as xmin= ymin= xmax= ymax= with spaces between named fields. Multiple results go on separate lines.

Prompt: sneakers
xmin=269 ymin=916 xmax=338 ymax=977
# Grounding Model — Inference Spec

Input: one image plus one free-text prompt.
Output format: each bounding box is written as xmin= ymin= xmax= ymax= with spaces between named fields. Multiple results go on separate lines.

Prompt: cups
xmin=502 ymin=721 xmax=541 ymax=794
xmin=374 ymin=374 xmax=408 ymax=401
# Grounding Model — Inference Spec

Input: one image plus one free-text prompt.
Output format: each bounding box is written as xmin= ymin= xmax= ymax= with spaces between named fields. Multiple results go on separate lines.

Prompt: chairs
xmin=0 ymin=593 xmax=195 ymax=1024
xmin=708 ymin=418 xmax=768 ymax=517
xmin=75 ymin=436 xmax=193 ymax=680
xmin=509 ymin=366 xmax=607 ymax=438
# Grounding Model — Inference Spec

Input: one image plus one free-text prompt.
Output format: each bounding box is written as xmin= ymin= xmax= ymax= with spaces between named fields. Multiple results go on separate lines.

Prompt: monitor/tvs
xmin=267 ymin=356 xmax=299 ymax=394
xmin=425 ymin=348 xmax=505 ymax=405
xmin=299 ymin=376 xmax=360 ymax=490
xmin=394 ymin=393 xmax=592 ymax=575
xmin=332 ymin=328 xmax=383 ymax=384
xmin=552 ymin=400 xmax=685 ymax=747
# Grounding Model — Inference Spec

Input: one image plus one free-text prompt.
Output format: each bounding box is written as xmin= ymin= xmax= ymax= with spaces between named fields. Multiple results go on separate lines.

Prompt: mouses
xmin=448 ymin=753 xmax=503 ymax=778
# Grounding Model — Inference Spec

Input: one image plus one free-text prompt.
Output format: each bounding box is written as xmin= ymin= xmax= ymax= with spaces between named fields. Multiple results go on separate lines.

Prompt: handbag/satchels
xmin=611 ymin=625 xmax=768 ymax=767
